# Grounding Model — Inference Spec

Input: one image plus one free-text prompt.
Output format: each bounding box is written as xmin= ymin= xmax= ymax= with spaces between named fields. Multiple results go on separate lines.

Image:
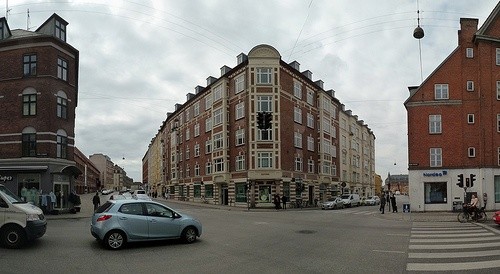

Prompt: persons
xmin=274 ymin=192 xmax=283 ymax=210
xmin=93 ymin=192 xmax=100 ymax=212
xmin=465 ymin=194 xmax=481 ymax=220
xmin=378 ymin=193 xmax=398 ymax=214
xmin=314 ymin=197 xmax=318 ymax=207
xmin=281 ymin=193 xmax=287 ymax=209
xmin=148 ymin=190 xmax=168 ymax=200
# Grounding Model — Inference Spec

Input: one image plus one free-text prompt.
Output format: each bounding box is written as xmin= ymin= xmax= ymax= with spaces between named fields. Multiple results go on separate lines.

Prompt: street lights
xmin=388 ymin=162 xmax=397 ymax=212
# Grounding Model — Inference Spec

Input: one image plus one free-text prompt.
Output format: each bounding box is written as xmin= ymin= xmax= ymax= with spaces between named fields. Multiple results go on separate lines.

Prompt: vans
xmin=341 ymin=194 xmax=361 ymax=207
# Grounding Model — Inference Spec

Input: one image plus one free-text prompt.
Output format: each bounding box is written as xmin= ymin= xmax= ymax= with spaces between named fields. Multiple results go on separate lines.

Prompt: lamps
xmin=413 ymin=9 xmax=424 ymax=39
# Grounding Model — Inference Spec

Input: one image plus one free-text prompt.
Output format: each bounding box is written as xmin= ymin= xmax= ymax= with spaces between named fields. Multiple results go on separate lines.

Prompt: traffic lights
xmin=249 ymin=180 xmax=252 ymax=189
xmin=256 ymin=111 xmax=264 ymax=130
xmin=264 ymin=113 xmax=272 ymax=129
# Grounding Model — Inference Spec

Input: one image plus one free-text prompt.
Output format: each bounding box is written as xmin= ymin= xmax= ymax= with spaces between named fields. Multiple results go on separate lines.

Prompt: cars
xmin=90 ymin=198 xmax=204 ymax=250
xmin=102 ymin=189 xmax=114 ymax=194
xmin=360 ymin=196 xmax=380 ymax=205
xmin=131 ymin=189 xmax=147 ymax=198
xmin=0 ymin=185 xmax=47 ymax=249
xmin=322 ymin=196 xmax=345 ymax=210
xmin=110 ymin=195 xmax=127 ymax=200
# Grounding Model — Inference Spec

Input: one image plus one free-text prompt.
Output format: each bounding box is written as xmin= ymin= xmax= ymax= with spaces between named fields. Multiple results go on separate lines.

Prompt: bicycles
xmin=456 ymin=202 xmax=489 ymax=224
